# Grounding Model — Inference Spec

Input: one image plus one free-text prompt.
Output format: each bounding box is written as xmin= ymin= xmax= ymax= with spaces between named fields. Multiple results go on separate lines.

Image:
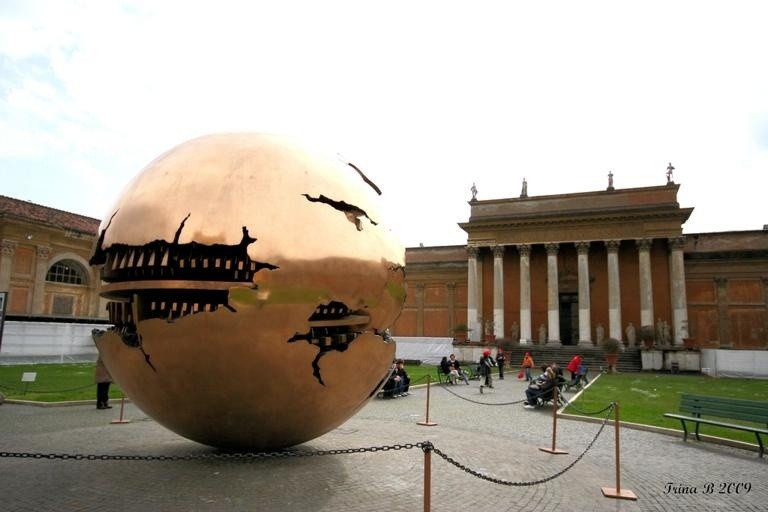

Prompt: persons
xmin=520 ymin=351 xmax=534 ymax=381
xmin=95 ymin=357 xmax=111 ymax=410
xmin=524 ymin=362 xmax=562 ymax=409
xmin=449 ymin=354 xmax=471 ymax=386
xmin=478 ymin=352 xmax=492 ymax=392
xmin=485 ymin=350 xmax=494 ymax=388
xmin=566 ymin=354 xmax=584 ymax=379
xmin=378 ymin=360 xmax=414 ymax=400
xmin=496 ymin=349 xmax=506 ymax=381
xmin=594 ymin=322 xmax=604 ymax=344
xmin=538 ymin=323 xmax=547 ymax=344
xmin=441 ymin=356 xmax=461 ymax=385
xmin=656 ymin=317 xmax=662 ymax=345
xmin=625 ymin=321 xmax=637 ymax=349
xmin=510 ymin=321 xmax=520 ymax=341
xmin=663 ymin=321 xmax=671 ymax=347
xmin=485 ymin=319 xmax=492 ymax=335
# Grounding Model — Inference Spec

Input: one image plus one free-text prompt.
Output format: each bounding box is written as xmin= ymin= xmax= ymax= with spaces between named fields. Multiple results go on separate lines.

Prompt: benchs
xmin=662 ymin=394 xmax=768 ymax=458
xmin=437 ymin=364 xmax=480 ymax=385
xmin=535 ymin=367 xmax=589 ymax=407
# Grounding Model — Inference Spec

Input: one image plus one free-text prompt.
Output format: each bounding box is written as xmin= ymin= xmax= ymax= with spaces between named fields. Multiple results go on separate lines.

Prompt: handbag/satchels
xmin=517 ymin=373 xmax=524 ymax=378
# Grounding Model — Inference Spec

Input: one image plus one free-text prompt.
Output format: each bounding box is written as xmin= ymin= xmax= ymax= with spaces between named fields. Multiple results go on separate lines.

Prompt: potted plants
xmin=496 ymin=337 xmax=519 ymax=369
xmin=638 ymin=324 xmax=659 ymax=346
xmin=603 ymin=337 xmax=620 ymax=375
xmin=448 ymin=323 xmax=475 ymax=342
xmin=680 ymin=316 xmax=704 ymax=348
xmin=485 ymin=319 xmax=496 ymax=346
